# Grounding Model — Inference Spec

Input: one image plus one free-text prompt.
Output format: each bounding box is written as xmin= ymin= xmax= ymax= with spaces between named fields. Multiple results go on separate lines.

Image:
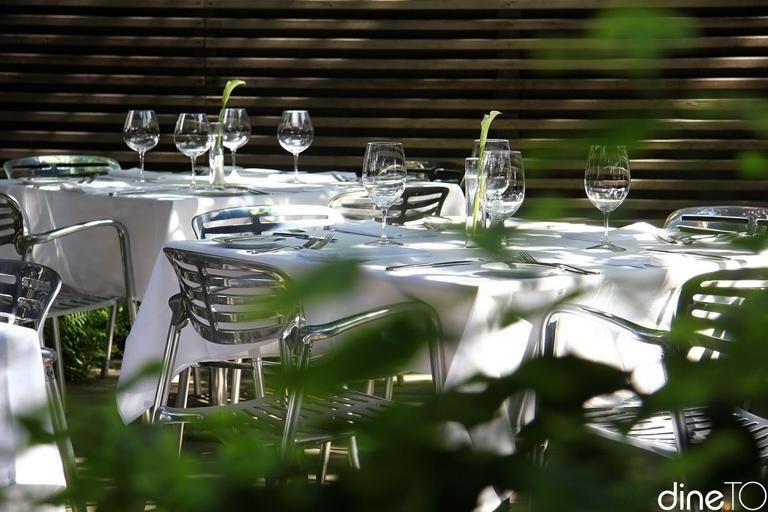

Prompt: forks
xmin=512 ymin=255 xmax=591 ymax=275
xmin=311 ymin=233 xmax=335 ymax=250
xmin=653 ymin=233 xmax=731 ymax=246
xmin=519 ymin=250 xmax=600 ymax=274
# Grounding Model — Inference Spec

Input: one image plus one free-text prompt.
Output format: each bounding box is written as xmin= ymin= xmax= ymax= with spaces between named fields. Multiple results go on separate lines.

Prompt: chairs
xmin=536 ymin=267 xmax=767 ymax=486
xmin=662 ymin=205 xmax=767 ymax=238
xmin=149 ymin=246 xmax=447 ymax=498
xmin=0 ymin=259 xmax=88 ymax=512
xmin=2 ymin=154 xmax=123 ymax=377
xmin=322 ymin=188 xmax=449 ymax=404
xmin=369 ymin=168 xmax=467 ymax=189
xmin=1 ymin=189 xmax=136 ymax=427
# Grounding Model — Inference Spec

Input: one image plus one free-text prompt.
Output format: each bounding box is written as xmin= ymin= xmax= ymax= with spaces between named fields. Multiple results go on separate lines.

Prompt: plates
xmin=481 ymin=262 xmax=558 ymax=279
xmin=309 ymin=236 xmax=337 ymax=240
xmin=18 ymin=177 xmax=65 ymax=184
xmin=212 ymin=236 xmax=295 ymax=248
xmin=274 ymin=228 xmax=306 ymax=234
xmin=70 ymin=180 xmax=126 ymax=189
xmin=296 ymin=235 xmax=308 ymax=239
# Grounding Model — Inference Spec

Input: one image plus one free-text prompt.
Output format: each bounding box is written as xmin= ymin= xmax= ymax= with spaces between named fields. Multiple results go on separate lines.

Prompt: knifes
xmin=386 ymin=260 xmax=473 ymax=271
xmin=646 ymin=248 xmax=731 ymax=261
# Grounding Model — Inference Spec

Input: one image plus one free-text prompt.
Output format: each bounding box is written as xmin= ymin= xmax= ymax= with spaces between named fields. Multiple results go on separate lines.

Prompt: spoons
xmin=668 ymin=236 xmax=715 ymax=241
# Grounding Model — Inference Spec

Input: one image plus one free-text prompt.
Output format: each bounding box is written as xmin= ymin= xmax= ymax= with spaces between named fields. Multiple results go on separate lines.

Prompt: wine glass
xmin=123 ymin=109 xmax=160 ymax=187
xmin=583 ymin=143 xmax=630 ymax=252
xmin=471 ymin=139 xmax=511 ymax=246
xmin=277 ymin=109 xmax=314 ymax=185
xmin=174 ymin=113 xmax=214 ymax=192
xmin=362 ymin=141 xmax=408 ymax=247
xmin=219 ymin=108 xmax=251 ymax=184
xmin=477 ymin=151 xmax=525 ymax=263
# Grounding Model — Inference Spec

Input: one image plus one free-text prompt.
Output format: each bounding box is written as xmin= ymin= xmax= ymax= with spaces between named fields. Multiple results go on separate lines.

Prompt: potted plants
xmin=206 ymin=79 xmax=246 ymax=188
xmin=464 ymin=111 xmax=503 ymax=239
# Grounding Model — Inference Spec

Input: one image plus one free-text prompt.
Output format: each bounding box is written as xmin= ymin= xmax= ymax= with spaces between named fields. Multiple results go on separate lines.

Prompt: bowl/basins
xmin=421 ymin=216 xmax=453 ymax=232
